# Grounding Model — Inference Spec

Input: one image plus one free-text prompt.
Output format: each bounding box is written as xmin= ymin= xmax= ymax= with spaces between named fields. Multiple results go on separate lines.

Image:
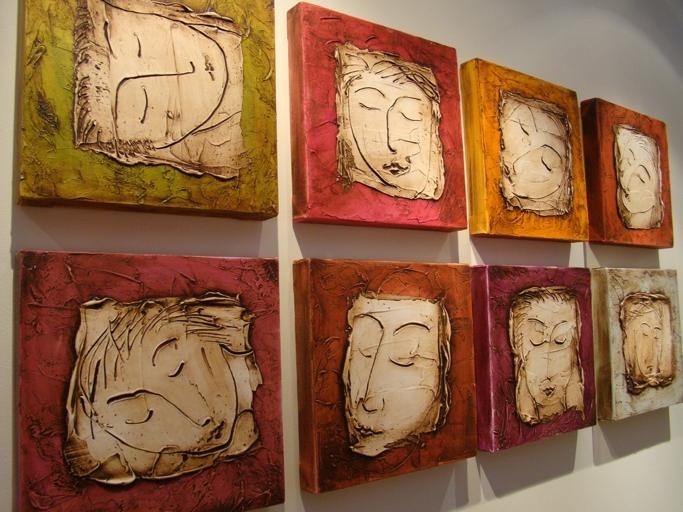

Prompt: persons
xmin=506 ymin=284 xmax=589 ymax=426
xmin=332 ymin=40 xmax=447 ymax=204
xmin=69 ymin=1 xmax=245 ymax=182
xmin=495 ymin=86 xmax=576 ymax=218
xmin=339 ymin=288 xmax=453 ymax=460
xmin=60 ymin=288 xmax=264 ymax=491
xmin=618 ymin=288 xmax=676 ymax=396
xmin=611 ymin=121 xmax=668 ymax=232
